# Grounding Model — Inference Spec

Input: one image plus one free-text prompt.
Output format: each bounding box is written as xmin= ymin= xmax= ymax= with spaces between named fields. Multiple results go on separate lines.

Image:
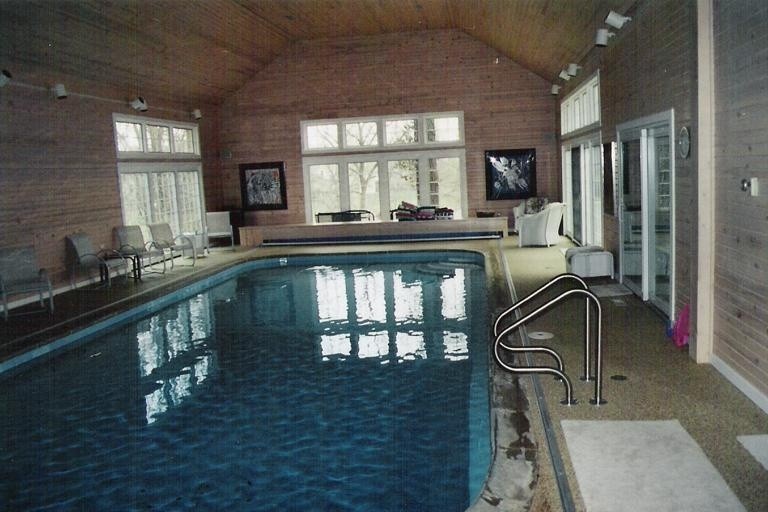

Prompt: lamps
xmin=594 ymin=8 xmax=632 ymax=47
xmin=133 ymin=96 xmax=150 ymax=112
xmin=53 ymin=83 xmax=67 ymax=100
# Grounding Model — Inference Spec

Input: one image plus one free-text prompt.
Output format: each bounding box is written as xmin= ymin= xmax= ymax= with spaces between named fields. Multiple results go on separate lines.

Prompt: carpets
xmin=559 ymin=411 xmax=768 ymax=510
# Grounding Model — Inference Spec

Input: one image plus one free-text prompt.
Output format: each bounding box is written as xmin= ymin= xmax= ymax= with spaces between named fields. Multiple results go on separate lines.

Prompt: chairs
xmin=0 ymin=244 xmax=57 ymax=324
xmin=513 ymin=195 xmax=565 ymax=247
xmin=66 ymin=210 xmax=236 ymax=295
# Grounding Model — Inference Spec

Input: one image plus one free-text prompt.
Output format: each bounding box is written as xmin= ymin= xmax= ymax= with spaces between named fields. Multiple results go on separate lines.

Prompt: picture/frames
xmin=484 ymin=148 xmax=537 ymax=201
xmin=238 ymin=160 xmax=288 ymax=211
